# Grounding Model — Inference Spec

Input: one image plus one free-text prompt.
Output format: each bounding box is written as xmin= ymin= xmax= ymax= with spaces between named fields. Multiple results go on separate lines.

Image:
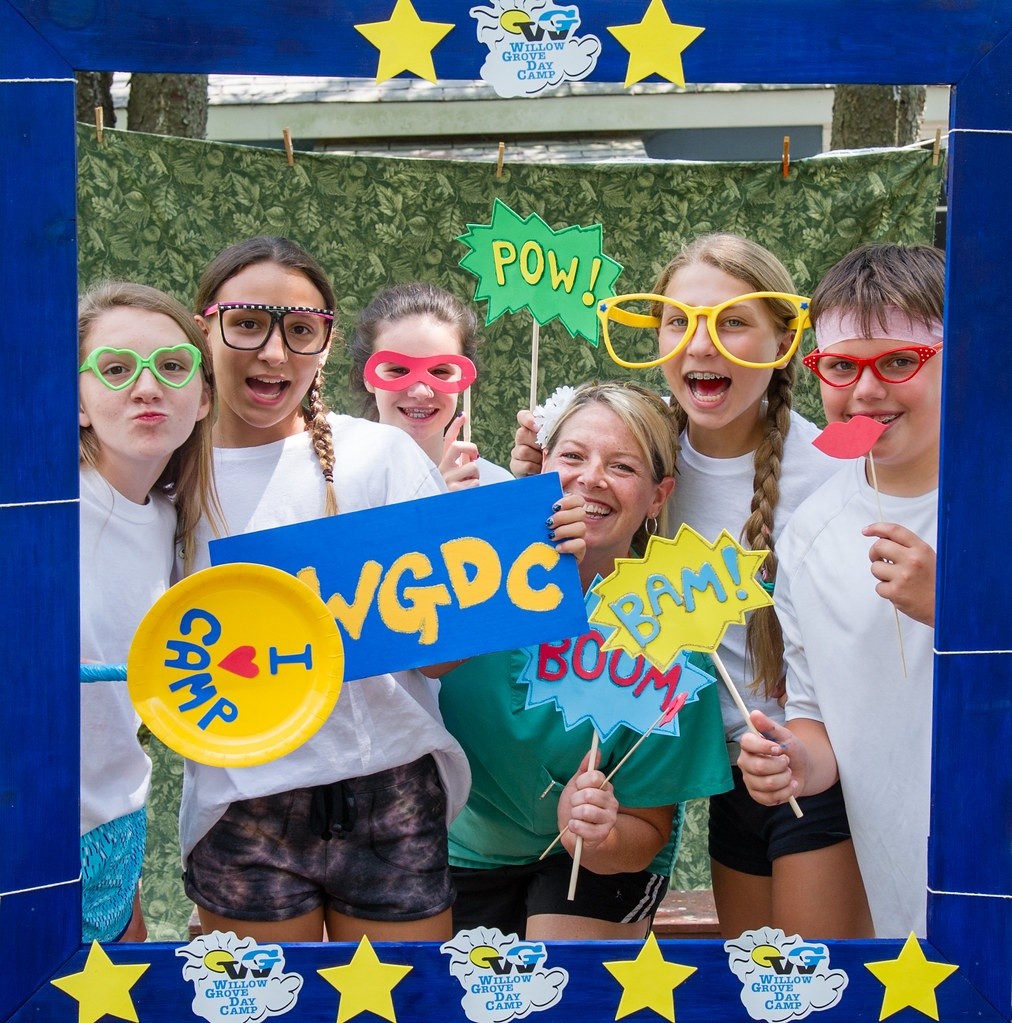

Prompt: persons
xmin=78 ymin=283 xmax=218 ymax=944
xmin=351 ymin=282 xmax=518 ymax=492
xmin=176 ymin=236 xmax=470 ymax=942
xmin=509 ymin=230 xmax=878 ymax=938
xmin=737 ymin=247 xmax=947 ymax=938
xmin=436 ymin=380 xmax=736 ymax=940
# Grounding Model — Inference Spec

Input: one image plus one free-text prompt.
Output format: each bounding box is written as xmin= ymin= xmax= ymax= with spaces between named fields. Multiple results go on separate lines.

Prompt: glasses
xmin=79 ymin=344 xmax=203 ymax=391
xmin=802 ymin=340 xmax=944 ymax=388
xmin=596 ymin=292 xmax=814 ymax=369
xmin=363 ymin=349 xmax=479 ymax=393
xmin=203 ymin=301 xmax=335 ymax=356
